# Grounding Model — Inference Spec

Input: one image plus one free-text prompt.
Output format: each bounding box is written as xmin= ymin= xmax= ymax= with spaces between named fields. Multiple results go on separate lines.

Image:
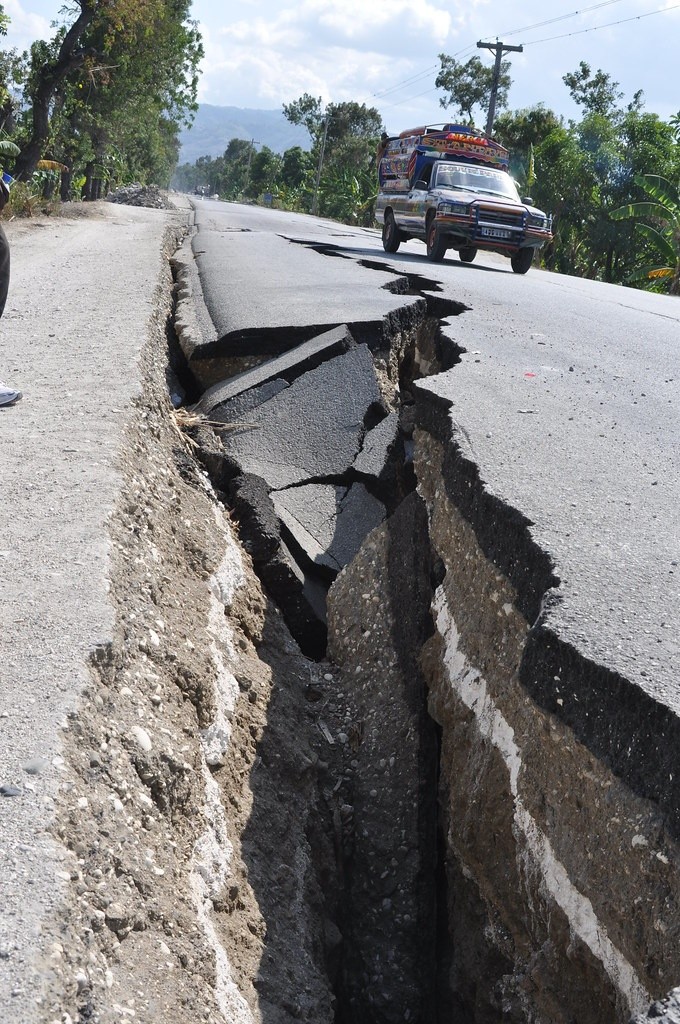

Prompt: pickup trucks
xmin=374 ymin=161 xmax=555 ymax=275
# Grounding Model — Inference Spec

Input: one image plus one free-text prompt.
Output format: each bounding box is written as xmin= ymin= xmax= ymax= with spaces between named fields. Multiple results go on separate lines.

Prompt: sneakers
xmin=0 ymin=382 xmax=23 ymax=406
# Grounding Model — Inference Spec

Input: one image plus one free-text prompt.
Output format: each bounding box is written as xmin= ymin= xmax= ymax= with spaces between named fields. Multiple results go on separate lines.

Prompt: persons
xmin=0 ymin=139 xmax=23 ymax=407
